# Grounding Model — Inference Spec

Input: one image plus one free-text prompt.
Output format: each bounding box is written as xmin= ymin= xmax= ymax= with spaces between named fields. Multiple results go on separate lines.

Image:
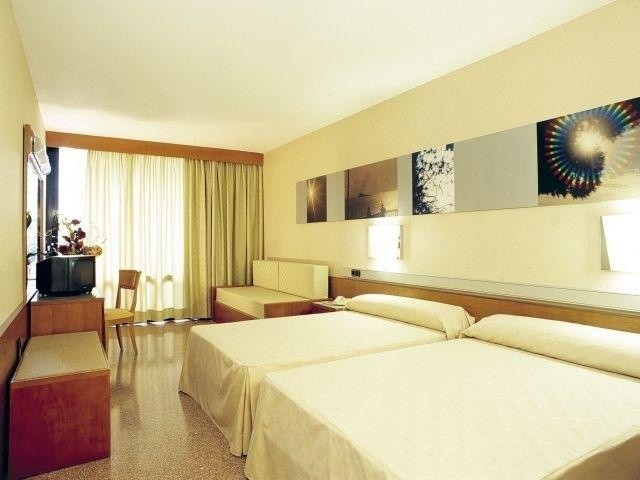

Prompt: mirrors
xmin=22 ymin=123 xmax=46 ymax=303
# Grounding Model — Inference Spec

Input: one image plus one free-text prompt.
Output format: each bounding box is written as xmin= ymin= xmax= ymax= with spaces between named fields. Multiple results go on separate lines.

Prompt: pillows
xmin=343 ymin=293 xmax=474 ymax=339
xmin=460 ymin=313 xmax=640 ymax=380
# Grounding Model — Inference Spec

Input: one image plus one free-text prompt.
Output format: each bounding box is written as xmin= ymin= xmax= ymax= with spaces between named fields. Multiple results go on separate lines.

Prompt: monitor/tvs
xmin=36 ymin=255 xmax=95 ymax=297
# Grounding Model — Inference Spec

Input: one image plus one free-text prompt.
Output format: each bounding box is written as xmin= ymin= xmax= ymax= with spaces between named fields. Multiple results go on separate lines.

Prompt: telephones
xmin=332 ymin=296 xmax=350 ymax=305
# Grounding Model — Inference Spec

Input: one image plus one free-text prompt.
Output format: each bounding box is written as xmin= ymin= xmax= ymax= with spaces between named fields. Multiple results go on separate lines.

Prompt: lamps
xmin=33 ymin=136 xmax=51 ymax=175
xmin=367 ymin=224 xmax=401 ymax=260
xmin=600 ymin=214 xmax=639 ymax=272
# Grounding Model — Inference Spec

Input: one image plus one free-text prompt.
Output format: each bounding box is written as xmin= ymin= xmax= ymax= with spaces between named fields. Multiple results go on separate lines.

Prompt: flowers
xmin=58 ymin=213 xmax=103 ymax=257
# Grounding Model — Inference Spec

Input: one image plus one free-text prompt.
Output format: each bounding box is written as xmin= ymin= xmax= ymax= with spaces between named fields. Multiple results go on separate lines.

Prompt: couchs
xmin=210 ymin=258 xmax=328 ymax=323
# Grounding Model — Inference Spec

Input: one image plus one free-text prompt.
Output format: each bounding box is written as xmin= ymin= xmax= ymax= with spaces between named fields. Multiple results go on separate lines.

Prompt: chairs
xmin=103 ymin=269 xmax=141 ymax=357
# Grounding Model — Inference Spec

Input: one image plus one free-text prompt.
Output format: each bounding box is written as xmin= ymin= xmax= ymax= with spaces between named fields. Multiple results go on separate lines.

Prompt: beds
xmin=243 ymin=337 xmax=640 ymax=480
xmin=176 ymin=310 xmax=474 ymax=456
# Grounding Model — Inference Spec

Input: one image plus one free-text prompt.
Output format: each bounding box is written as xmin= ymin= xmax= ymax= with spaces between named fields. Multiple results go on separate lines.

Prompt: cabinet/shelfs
xmin=32 ymin=288 xmax=105 ymax=355
xmin=6 ymin=330 xmax=111 ymax=480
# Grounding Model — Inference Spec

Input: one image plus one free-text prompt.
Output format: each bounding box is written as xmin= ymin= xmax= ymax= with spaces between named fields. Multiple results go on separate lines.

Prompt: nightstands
xmin=311 ymin=300 xmax=346 ymax=313
